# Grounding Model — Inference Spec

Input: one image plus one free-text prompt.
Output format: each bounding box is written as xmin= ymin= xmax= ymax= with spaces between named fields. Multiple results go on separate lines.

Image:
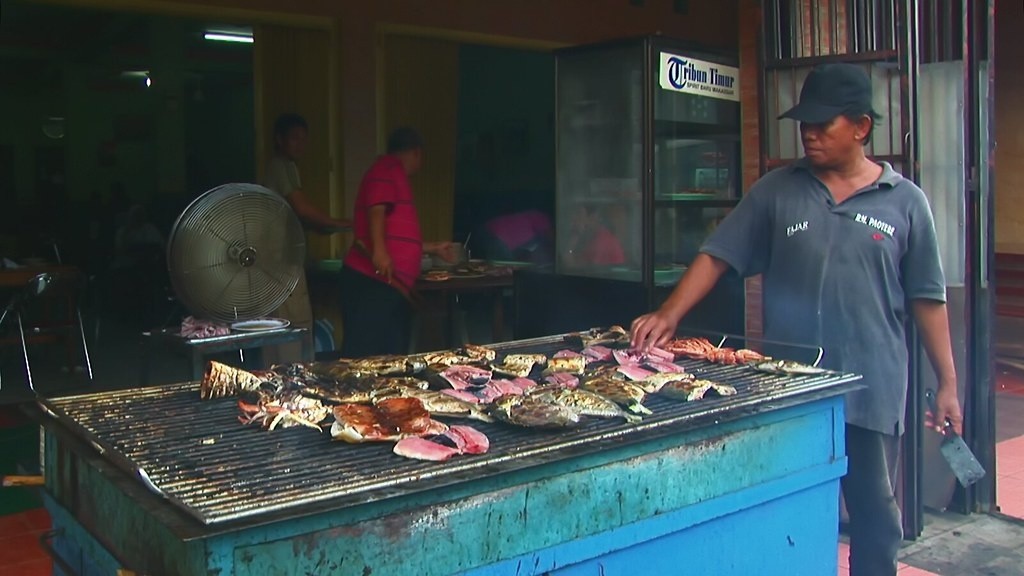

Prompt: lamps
xmin=203 ymin=32 xmax=254 ymax=43
xmin=146 ymin=71 xmax=161 ymax=88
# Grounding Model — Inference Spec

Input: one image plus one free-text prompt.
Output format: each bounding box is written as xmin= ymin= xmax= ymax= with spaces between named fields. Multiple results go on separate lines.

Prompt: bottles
xmin=420 ymin=253 xmax=432 ymax=271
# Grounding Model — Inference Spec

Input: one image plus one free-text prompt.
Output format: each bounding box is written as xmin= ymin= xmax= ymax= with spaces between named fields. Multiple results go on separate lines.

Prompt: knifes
xmin=924 ymin=388 xmax=985 ymax=487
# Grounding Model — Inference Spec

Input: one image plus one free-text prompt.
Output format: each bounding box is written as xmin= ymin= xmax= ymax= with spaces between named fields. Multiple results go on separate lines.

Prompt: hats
xmin=776 ymin=63 xmax=872 ymax=123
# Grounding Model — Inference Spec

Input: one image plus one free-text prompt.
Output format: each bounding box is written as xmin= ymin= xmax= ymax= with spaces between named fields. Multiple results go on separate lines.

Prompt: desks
xmin=404 ymin=261 xmax=521 ymax=355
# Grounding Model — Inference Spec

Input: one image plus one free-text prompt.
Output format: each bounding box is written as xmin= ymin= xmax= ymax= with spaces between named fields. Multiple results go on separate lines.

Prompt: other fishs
xmin=581 ymin=374 xmax=653 ymax=415
xmin=314 ymin=344 xmax=495 ymax=376
xmin=746 ymin=358 xmax=842 ymax=376
xmin=485 ymin=391 xmax=579 ymax=427
xmin=530 ymin=385 xmax=645 ymax=425
xmin=302 ymin=374 xmax=495 ymax=425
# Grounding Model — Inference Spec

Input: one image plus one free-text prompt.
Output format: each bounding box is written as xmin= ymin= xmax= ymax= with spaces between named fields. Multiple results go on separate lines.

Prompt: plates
xmin=229 ymin=317 xmax=290 ymax=332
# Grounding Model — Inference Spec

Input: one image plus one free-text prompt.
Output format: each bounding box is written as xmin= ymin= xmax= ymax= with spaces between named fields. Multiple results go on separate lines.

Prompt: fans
xmin=164 ymin=182 xmax=307 ymax=324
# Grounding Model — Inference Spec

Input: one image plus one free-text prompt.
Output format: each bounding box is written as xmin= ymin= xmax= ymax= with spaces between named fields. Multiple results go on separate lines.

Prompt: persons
xmin=341 ymin=127 xmax=457 ymax=359
xmin=470 ymin=206 xmax=625 ymax=326
xmin=259 ymin=113 xmax=354 ymax=367
xmin=629 ymin=65 xmax=962 ymax=576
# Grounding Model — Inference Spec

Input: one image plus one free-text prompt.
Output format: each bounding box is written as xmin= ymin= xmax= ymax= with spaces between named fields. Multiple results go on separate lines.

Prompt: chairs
xmin=0 ymin=263 xmax=95 ymax=393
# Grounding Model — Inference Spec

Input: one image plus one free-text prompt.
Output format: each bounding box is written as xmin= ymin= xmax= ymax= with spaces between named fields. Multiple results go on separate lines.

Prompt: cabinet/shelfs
xmin=549 ymin=35 xmax=741 ymax=297
